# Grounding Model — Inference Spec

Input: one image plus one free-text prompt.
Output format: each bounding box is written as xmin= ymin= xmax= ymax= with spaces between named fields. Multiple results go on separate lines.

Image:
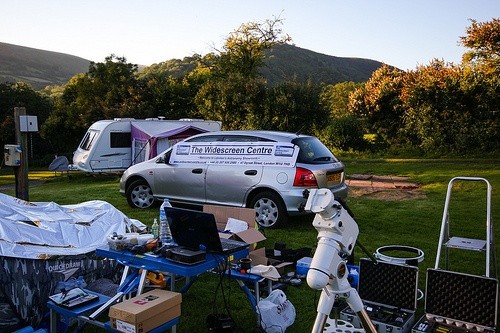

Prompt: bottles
xmin=55 ymin=276 xmax=87 ymax=324
xmin=152 ymin=218 xmax=159 ymax=238
xmin=159 ymin=199 xmax=172 ymax=240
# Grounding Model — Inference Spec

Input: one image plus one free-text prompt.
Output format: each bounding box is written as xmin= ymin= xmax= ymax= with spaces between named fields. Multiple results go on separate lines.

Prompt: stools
xmin=47 ymin=287 xmax=179 ymax=333
xmin=216 ymin=262 xmax=274 ymax=328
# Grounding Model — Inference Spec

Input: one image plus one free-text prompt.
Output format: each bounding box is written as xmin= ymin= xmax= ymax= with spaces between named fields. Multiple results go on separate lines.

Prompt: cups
xmin=237 ymin=258 xmax=252 ymax=271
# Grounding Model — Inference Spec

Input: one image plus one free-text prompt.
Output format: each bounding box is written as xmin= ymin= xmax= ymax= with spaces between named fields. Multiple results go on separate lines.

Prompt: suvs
xmin=119 ymin=128 xmax=348 ymax=227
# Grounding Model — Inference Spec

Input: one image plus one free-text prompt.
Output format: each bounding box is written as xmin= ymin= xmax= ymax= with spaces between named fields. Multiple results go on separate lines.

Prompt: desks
xmin=95 ymin=232 xmax=262 ymax=333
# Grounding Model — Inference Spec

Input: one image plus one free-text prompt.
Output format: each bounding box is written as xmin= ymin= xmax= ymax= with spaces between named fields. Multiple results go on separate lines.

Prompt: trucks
xmin=48 ymin=118 xmax=223 ymax=173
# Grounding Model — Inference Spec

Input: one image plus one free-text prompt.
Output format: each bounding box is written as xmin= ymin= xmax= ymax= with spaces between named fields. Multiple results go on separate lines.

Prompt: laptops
xmin=164 ymin=207 xmax=250 ymax=254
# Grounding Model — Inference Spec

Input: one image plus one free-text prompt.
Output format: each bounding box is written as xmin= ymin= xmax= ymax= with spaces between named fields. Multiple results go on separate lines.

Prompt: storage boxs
xmin=109 ymin=289 xmax=183 ymax=333
xmin=405 ymin=265 xmax=498 ymax=333
xmin=339 ymin=257 xmax=420 ymax=333
xmin=248 ymin=247 xmax=294 ymax=275
xmin=200 ymin=203 xmax=267 ymax=253
